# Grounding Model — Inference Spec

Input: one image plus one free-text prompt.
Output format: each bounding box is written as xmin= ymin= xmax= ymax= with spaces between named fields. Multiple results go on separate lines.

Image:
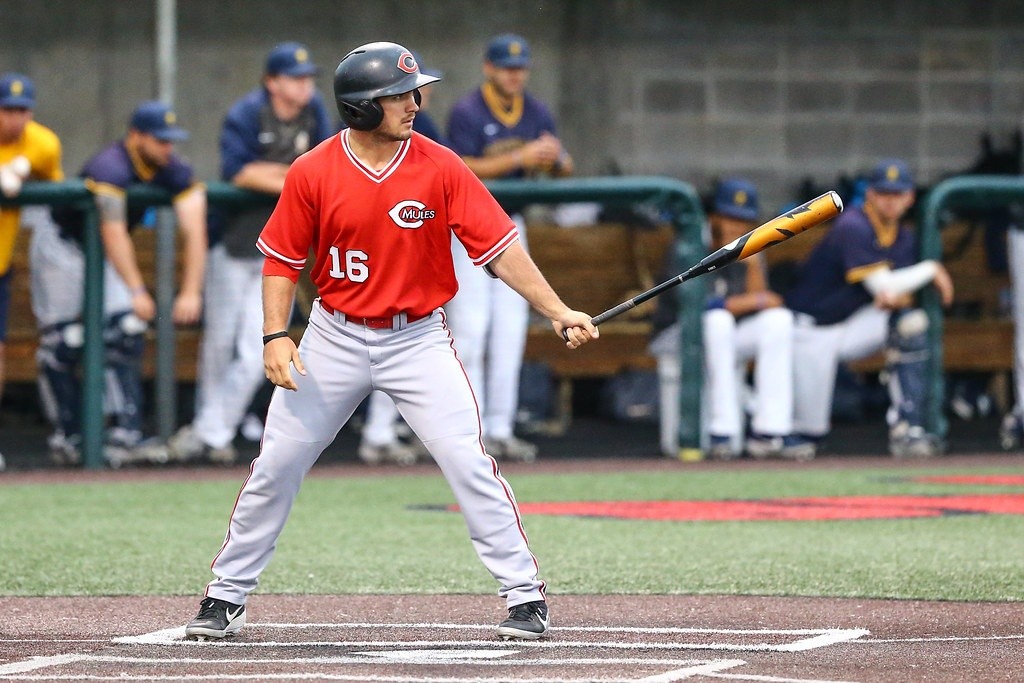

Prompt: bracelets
xmin=263 ymin=331 xmax=288 ymax=345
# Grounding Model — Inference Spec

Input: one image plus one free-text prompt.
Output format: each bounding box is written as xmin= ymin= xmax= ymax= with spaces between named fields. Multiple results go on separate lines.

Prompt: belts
xmin=317 ymin=298 xmax=427 ymax=329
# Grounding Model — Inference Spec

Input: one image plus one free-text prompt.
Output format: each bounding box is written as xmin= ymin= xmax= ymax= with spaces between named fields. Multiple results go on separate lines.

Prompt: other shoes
xmin=482 ymin=433 xmax=540 ymax=460
xmin=780 ymin=440 xmax=818 ymax=460
xmin=168 ymin=426 xmax=204 ymax=461
xmin=357 ymin=440 xmax=419 ymax=464
xmin=207 ymin=444 xmax=239 ymax=463
xmin=890 ymin=432 xmax=938 ymax=458
xmin=102 ymin=442 xmax=175 ymax=463
xmin=708 ymin=443 xmax=733 ymax=462
xmin=745 ymin=438 xmax=783 ymax=460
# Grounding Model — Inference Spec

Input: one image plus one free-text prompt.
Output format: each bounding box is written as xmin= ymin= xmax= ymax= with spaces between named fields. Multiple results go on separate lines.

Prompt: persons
xmin=167 ymin=35 xmax=574 ymax=467
xmin=650 ymin=131 xmax=1024 ymax=460
xmin=0 ymin=73 xmax=206 ymax=468
xmin=186 ymin=41 xmax=599 ymax=643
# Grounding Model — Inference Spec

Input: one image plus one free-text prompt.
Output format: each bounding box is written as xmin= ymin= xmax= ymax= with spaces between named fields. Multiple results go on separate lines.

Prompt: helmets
xmin=334 ymin=41 xmax=440 ymax=131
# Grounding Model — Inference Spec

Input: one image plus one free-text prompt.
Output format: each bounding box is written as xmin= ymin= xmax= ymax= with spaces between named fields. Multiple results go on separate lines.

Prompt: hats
xmin=264 ymin=41 xmax=314 ymax=76
xmin=712 ymin=181 xmax=760 ymax=219
xmin=0 ymin=74 xmax=33 ymax=107
xmin=130 ymin=102 xmax=187 ymax=140
xmin=870 ymin=159 xmax=913 ymax=193
xmin=485 ymin=33 xmax=530 ymax=68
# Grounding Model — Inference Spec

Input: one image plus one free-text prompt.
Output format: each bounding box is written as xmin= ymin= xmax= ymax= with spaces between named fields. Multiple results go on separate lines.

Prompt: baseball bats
xmin=562 ymin=190 xmax=844 ymax=342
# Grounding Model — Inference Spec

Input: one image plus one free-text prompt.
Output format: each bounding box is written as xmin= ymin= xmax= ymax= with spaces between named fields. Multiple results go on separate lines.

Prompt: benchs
xmin=0 ymin=225 xmax=1014 ymax=436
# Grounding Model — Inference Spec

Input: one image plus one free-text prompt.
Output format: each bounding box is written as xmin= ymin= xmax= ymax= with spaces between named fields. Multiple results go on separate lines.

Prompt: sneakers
xmin=498 ymin=601 xmax=550 ymax=640
xmin=186 ymin=598 xmax=246 ymax=637
